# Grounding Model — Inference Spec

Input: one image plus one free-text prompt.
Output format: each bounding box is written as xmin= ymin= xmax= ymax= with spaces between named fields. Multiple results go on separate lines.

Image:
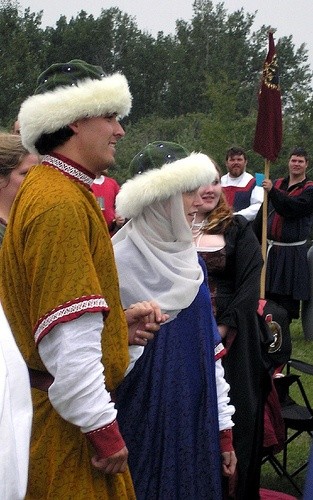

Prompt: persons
xmin=0 ymin=119 xmax=313 ymax=500
xmin=0 ymin=59 xmax=169 ymax=500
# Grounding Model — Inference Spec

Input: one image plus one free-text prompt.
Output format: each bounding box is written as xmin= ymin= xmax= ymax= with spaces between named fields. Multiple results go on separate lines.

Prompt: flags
xmin=253 ymin=32 xmax=282 ymax=164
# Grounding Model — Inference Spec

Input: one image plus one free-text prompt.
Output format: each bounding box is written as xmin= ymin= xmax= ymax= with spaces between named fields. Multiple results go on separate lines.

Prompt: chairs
xmin=263 ymin=302 xmax=313 ymax=496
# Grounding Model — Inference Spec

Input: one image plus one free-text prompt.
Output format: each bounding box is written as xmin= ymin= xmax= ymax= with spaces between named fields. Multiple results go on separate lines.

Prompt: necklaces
xmin=191 ymin=213 xmax=208 ymax=248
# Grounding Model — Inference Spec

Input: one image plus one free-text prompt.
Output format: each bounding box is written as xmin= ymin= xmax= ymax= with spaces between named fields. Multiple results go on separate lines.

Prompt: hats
xmin=116 ymin=141 xmax=217 ymax=220
xmin=18 ymin=59 xmax=132 ymax=154
